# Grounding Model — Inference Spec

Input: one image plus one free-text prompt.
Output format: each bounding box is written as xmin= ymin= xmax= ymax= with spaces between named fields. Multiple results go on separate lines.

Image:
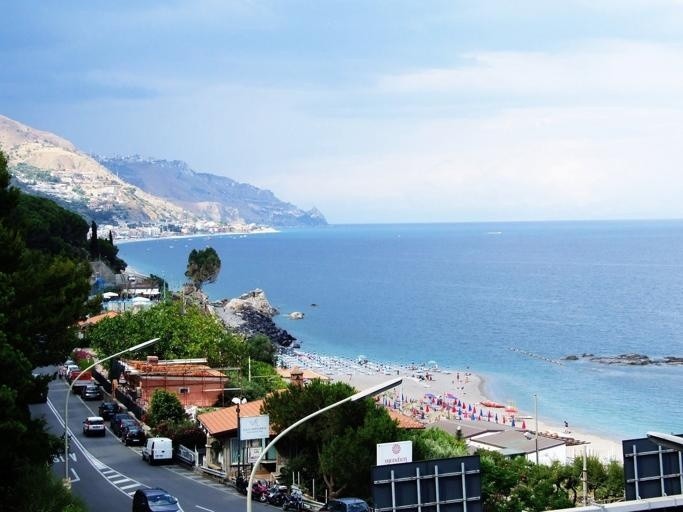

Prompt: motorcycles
xmin=236 ymin=472 xmax=311 ymax=512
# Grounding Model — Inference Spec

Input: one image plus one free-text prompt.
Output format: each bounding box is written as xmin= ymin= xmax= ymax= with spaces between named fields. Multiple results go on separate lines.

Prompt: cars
xmin=57 ymin=358 xmax=104 ymax=401
xmin=98 ymin=399 xmax=144 ymax=447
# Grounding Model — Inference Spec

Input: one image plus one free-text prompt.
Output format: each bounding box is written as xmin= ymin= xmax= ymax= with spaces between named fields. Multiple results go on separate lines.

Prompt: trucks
xmin=140 ymin=437 xmax=173 ymax=465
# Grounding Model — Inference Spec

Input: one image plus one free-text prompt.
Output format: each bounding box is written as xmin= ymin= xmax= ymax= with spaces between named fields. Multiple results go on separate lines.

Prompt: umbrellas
xmin=381 ymin=389 xmax=527 ymax=431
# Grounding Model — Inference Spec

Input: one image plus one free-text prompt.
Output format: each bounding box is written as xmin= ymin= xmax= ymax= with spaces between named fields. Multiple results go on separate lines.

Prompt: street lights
xmin=245 ymin=376 xmax=404 ymax=512
xmin=61 ymin=337 xmax=162 ymax=490
xmin=531 ymin=393 xmax=539 ymax=466
xmin=231 ymin=393 xmax=248 ymax=479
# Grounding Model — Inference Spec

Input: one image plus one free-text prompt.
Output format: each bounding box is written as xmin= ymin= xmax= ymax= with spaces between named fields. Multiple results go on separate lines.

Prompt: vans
xmin=314 ymin=497 xmax=369 ymax=511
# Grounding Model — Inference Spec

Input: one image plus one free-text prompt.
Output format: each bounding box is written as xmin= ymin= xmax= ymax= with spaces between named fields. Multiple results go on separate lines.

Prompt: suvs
xmin=80 ymin=415 xmax=106 ymax=437
xmin=130 ymin=486 xmax=183 ymax=512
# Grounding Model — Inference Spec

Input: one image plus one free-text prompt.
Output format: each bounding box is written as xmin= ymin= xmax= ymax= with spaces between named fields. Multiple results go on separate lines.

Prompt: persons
xmin=410 ymin=362 xmax=471 ymax=395
xmin=563 ymin=420 xmax=569 ymax=433
